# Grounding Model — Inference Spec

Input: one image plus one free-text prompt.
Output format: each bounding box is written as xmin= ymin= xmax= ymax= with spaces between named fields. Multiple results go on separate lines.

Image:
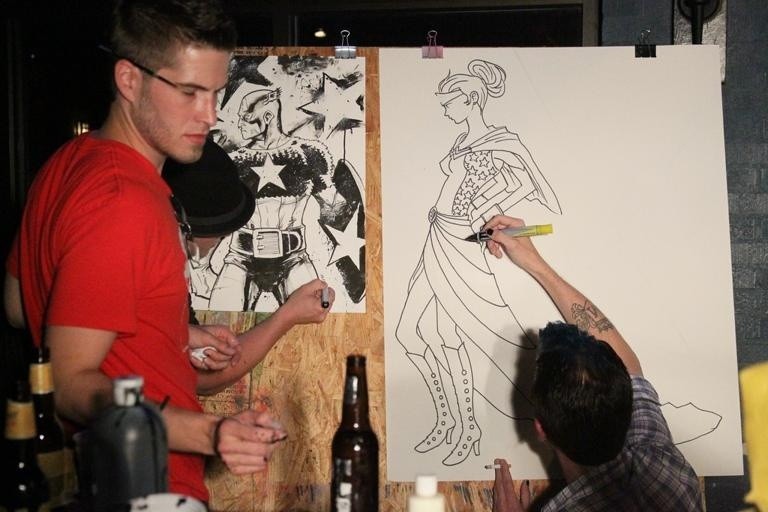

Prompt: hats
xmin=161 ymin=138 xmax=256 ymax=238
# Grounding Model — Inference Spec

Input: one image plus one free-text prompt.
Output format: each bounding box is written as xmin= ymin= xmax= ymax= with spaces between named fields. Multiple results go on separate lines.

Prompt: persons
xmin=162 ymin=139 xmax=335 ymax=399
xmin=0 ymin=0 xmax=281 ymax=512
xmin=481 ymin=214 xmax=707 ymax=512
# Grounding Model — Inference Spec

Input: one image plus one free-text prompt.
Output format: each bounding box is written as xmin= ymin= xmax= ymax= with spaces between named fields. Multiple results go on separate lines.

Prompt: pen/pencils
xmin=321 ymin=280 xmax=329 ymax=308
xmin=465 ymin=224 xmax=553 ymax=241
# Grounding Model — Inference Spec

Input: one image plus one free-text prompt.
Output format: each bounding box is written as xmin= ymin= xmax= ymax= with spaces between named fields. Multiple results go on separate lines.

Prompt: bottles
xmin=0 ymin=345 xmax=169 ymax=512
xmin=406 ymin=477 xmax=444 ymax=512
xmin=330 ymin=354 xmax=380 ymax=512
